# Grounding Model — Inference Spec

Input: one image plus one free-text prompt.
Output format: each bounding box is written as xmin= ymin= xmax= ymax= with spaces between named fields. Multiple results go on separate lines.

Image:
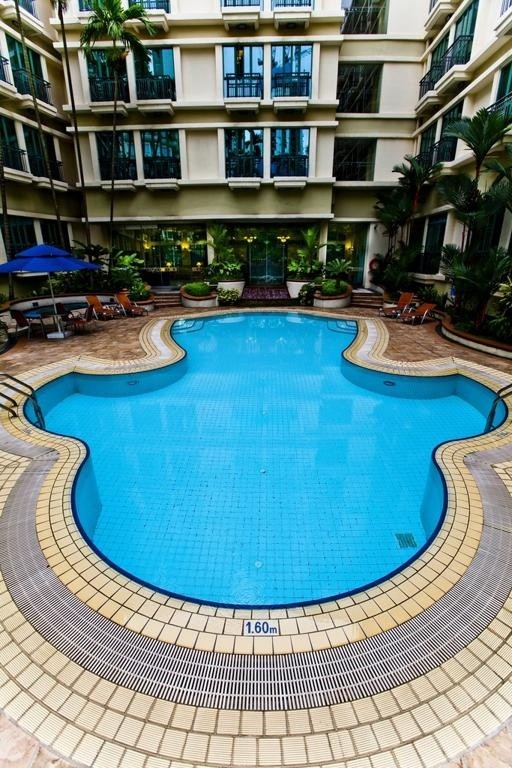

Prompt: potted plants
xmin=194 ymin=224 xmax=246 ymax=298
xmin=285 ymin=226 xmax=337 ymax=299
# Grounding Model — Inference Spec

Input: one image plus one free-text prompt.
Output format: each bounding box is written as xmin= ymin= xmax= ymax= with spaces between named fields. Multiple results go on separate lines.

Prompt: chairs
xmin=114 ymin=293 xmax=147 ymax=318
xmin=9 ymin=302 xmax=96 ymax=341
xmin=382 ymin=291 xmax=436 ymax=327
xmin=85 ymin=296 xmax=120 ymax=321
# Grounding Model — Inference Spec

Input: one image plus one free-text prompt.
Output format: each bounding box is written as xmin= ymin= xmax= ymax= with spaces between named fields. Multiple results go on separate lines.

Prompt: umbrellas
xmin=0 ymin=242 xmax=105 ymax=332
